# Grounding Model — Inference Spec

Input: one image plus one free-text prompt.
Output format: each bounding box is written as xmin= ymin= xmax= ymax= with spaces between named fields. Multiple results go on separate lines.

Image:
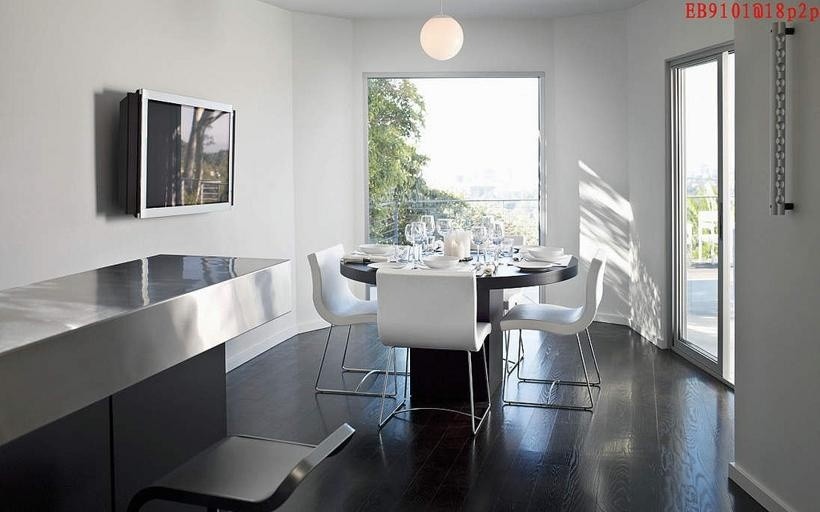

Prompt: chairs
xmin=376 ymin=267 xmax=493 ymax=436
xmin=308 ymin=243 xmax=410 ymax=398
xmin=496 ymin=242 xmax=609 ymax=411
xmin=504 ymin=235 xmax=526 ymax=377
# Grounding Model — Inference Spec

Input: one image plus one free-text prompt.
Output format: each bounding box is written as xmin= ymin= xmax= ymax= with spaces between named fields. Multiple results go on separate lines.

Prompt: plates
xmin=405 ymin=215 xmax=453 ymax=270
xmin=361 ymin=245 xmax=391 ymax=254
xmin=367 ymin=262 xmax=406 ymax=269
xmin=512 ymin=246 xmax=574 ymax=269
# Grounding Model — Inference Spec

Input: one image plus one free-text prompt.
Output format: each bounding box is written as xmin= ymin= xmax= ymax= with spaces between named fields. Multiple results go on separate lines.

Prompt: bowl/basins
xmin=422 ymin=255 xmax=459 ymax=268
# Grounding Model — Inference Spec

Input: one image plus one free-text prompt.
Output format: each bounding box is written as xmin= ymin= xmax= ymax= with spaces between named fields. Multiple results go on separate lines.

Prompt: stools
xmin=125 ymin=423 xmax=360 ymax=510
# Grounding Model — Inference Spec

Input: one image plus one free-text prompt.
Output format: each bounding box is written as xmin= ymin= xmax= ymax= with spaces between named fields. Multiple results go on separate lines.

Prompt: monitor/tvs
xmin=119 ymin=87 xmax=236 ymax=220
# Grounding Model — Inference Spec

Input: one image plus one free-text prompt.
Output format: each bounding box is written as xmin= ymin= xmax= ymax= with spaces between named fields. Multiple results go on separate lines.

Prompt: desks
xmin=338 ymin=245 xmax=579 ymax=417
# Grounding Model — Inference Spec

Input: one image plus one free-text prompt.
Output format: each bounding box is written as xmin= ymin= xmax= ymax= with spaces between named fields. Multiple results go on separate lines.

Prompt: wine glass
xmin=471 ymin=216 xmax=505 ymax=261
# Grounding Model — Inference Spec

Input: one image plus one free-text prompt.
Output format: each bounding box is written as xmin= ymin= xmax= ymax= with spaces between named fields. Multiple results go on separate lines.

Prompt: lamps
xmin=418 ymin=1 xmax=466 ymax=62
